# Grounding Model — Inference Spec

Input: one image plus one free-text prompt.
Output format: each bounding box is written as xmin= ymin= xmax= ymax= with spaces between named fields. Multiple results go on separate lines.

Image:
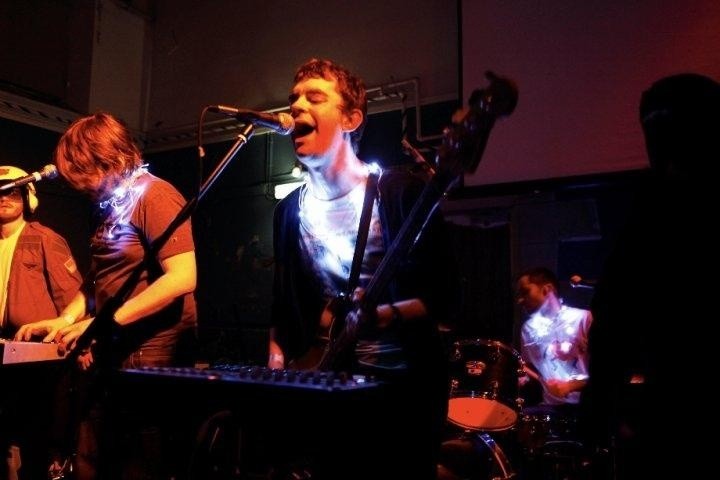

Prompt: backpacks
xmin=522 ymin=415 xmax=599 ymax=476
xmin=436 ymin=431 xmax=523 ymax=479
xmin=447 ymin=337 xmax=524 ymax=432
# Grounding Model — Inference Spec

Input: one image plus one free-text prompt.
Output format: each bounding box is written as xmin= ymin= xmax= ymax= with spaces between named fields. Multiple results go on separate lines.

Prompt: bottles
xmin=0 ymin=165 xmax=36 ymax=191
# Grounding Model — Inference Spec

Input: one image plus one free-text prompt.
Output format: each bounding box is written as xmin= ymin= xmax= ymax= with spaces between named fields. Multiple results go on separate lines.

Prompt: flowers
xmin=0 ymin=164 xmax=58 ymax=191
xmin=208 ymin=104 xmax=296 ymax=137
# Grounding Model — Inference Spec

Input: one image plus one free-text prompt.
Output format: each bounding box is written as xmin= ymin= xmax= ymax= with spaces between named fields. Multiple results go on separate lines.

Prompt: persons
xmin=0 ymin=164 xmax=94 ymax=480
xmin=10 ymin=110 xmax=198 ymax=371
xmin=265 ymin=56 xmax=464 ymax=480
xmin=577 ymin=70 xmax=720 ymax=480
xmin=511 ymin=263 xmax=593 ymax=418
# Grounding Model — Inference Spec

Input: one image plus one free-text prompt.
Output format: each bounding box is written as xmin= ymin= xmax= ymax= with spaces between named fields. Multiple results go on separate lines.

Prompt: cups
xmin=1 ymin=188 xmax=16 ymax=196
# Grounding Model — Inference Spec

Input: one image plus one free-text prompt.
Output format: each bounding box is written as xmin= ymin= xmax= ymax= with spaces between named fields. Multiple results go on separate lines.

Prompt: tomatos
xmin=0 ymin=337 xmax=72 ymax=365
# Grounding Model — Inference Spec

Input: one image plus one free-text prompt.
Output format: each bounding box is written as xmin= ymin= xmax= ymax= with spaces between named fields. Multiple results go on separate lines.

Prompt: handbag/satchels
xmin=389 ymin=300 xmax=404 ymax=326
